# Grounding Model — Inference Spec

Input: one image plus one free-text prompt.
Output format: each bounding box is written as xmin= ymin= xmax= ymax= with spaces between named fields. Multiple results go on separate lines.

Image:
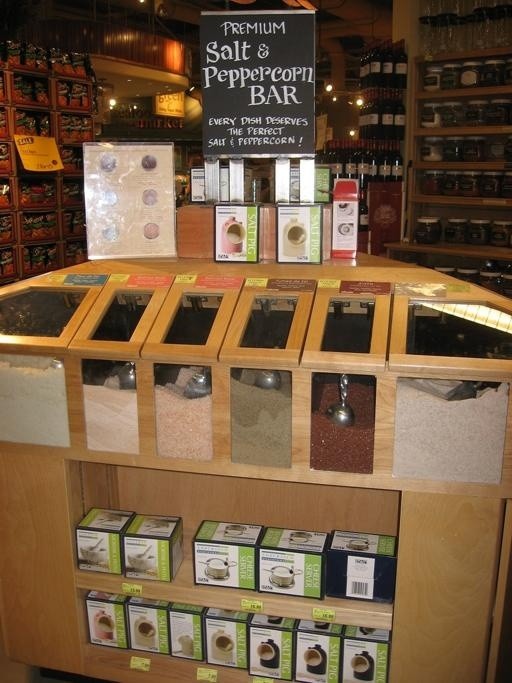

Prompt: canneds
xmin=408 ymin=58 xmax=512 ymax=300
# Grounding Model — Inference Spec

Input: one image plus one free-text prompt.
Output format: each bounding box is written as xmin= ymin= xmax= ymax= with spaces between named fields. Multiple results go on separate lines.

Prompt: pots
xmin=224 ymin=524 xmax=246 ymax=537
xmin=288 ymin=531 xmax=312 ymax=542
xmin=197 ymin=555 xmax=238 ymax=581
xmin=263 ymin=565 xmax=304 ymax=589
xmin=347 ymin=538 xmax=370 ymax=550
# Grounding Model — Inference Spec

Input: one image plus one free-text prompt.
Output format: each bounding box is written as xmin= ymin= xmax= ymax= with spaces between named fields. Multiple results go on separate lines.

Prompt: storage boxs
xmin=175 ymin=155 xmax=363 ymax=267
xmin=294 ymin=617 xmax=341 ymax=683
xmin=203 ymin=604 xmax=248 ymax=670
xmin=81 ymin=588 xmax=132 ymax=650
xmin=323 ymin=527 xmax=395 ymax=605
xmin=125 ymin=595 xmax=171 ymax=657
xmin=122 ymin=513 xmax=184 ymax=584
xmin=258 ymin=527 xmax=330 ymax=602
xmin=76 ymin=507 xmax=135 ymax=575
xmin=167 ymin=601 xmax=206 ymax=663
xmin=342 ymin=625 xmax=392 ymax=683
xmin=193 ymin=519 xmax=264 ymax=591
xmin=248 ymin=612 xmax=293 ymax=682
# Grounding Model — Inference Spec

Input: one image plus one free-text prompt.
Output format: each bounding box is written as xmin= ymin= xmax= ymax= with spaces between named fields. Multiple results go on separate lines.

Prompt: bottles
xmin=111 ymin=106 xmax=184 ymax=129
xmin=322 ymin=38 xmax=406 ymax=233
xmin=250 ymin=176 xmax=264 ymax=203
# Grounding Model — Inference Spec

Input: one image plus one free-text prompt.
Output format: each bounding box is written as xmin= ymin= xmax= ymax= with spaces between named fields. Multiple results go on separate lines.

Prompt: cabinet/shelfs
xmin=400 ymin=46 xmax=512 ymax=260
xmin=0 ymin=44 xmax=99 ymax=285
xmin=319 ymin=38 xmax=407 ymax=258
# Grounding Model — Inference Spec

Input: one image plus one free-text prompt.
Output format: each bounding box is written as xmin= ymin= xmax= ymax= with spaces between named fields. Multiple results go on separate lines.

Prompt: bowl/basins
xmin=128 ymin=544 xmax=157 ymax=574
xmin=80 ymin=538 xmax=109 ymax=568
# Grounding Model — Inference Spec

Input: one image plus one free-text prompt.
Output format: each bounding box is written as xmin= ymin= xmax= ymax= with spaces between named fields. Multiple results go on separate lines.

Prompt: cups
xmin=140 ymin=518 xmax=170 ymax=529
xmin=99 ymin=512 xmax=123 ymax=521
